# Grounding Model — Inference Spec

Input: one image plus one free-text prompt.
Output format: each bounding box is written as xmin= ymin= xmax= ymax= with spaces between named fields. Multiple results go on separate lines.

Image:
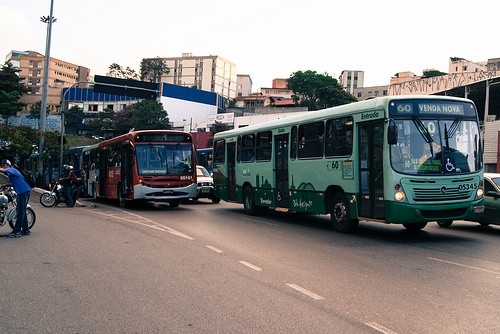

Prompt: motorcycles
xmin=40 ymin=180 xmax=77 ymax=207
xmin=0 ymin=186 xmax=36 ymax=230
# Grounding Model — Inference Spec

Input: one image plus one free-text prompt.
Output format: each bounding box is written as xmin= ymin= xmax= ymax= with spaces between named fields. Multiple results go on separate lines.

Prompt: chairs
xmin=392 ymin=145 xmax=417 ymax=170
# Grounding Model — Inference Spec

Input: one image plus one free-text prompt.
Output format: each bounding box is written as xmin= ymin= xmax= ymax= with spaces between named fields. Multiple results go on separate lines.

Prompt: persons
xmin=59 ymin=157 xmax=89 ymax=208
xmin=0 ymin=159 xmax=31 ymax=239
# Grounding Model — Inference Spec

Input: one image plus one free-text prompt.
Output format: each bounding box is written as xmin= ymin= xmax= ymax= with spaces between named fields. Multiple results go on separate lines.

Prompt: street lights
xmin=183 ymin=117 xmax=193 ymax=135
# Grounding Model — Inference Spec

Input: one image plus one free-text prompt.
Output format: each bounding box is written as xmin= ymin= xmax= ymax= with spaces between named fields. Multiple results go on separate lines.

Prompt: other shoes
xmin=68 ymin=204 xmax=73 ymax=207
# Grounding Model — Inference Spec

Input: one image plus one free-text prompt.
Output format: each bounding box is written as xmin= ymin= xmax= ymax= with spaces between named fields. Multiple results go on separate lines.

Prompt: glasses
xmin=1 ymin=165 xmax=8 ymax=167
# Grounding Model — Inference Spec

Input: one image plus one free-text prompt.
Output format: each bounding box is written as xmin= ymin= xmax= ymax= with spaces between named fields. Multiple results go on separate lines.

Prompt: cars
xmin=195 ymin=165 xmax=220 ymax=204
xmin=437 ymin=172 xmax=500 ymax=230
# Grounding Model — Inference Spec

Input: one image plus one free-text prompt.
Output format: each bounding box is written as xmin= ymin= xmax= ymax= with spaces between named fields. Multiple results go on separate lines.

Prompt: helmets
xmin=1 ymin=159 xmax=11 ymax=166
xmin=66 ymin=166 xmax=73 ymax=169
xmin=63 ymin=164 xmax=69 ymax=168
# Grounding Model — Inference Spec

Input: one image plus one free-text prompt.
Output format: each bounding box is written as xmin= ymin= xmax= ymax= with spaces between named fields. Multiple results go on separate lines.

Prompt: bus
xmin=79 ymin=127 xmax=198 ymax=208
xmin=211 ymin=94 xmax=486 ymax=233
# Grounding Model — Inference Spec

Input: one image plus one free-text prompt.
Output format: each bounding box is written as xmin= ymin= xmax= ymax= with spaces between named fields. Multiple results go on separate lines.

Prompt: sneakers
xmin=9 ymin=231 xmax=21 ymax=238
xmin=21 ymin=230 xmax=28 ymax=235
